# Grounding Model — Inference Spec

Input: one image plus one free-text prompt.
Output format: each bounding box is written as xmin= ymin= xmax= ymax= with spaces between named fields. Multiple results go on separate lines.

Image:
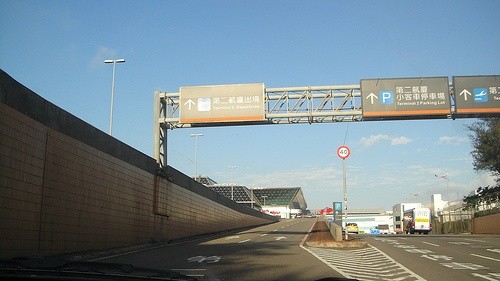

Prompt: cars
xmin=306 ymin=213 xmax=316 ymax=218
xmin=342 ymin=222 xmax=359 ymax=234
xmin=370 ymin=229 xmax=380 ymax=235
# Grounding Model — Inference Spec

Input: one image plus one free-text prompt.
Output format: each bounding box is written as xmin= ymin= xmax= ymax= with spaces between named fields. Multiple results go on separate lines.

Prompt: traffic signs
xmin=178 ymin=82 xmax=266 ymax=125
xmin=358 ymin=75 xmax=453 ymax=119
xmin=452 ymin=74 xmax=500 ymax=115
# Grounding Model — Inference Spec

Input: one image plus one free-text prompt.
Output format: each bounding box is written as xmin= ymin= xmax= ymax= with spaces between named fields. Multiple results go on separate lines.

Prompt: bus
xmin=401 ymin=207 xmax=432 ymax=234
xmin=378 ymin=223 xmax=390 ymax=234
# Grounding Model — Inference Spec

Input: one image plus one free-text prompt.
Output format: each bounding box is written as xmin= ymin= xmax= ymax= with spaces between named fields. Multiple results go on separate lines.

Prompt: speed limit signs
xmin=337 ymin=145 xmax=350 ymax=160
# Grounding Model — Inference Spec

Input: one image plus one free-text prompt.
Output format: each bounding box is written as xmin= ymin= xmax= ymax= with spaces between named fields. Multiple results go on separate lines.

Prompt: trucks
xmin=391 ymin=203 xmax=422 ymax=234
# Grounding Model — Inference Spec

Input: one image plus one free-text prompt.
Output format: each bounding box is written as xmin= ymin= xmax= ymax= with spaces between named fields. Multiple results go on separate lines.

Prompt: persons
xmin=410 ymin=221 xmax=415 ymax=234
xmin=407 ymin=221 xmax=411 ymax=234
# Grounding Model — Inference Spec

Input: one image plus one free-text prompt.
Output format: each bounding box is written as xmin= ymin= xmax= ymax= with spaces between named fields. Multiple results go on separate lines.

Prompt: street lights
xmin=435 ymin=174 xmax=452 ymax=234
xmin=227 ymin=165 xmax=238 ymax=201
xmin=190 ymin=133 xmax=204 ymax=181
xmin=262 ymin=195 xmax=268 ymax=213
xmin=248 ymin=184 xmax=256 ymax=208
xmin=104 ymin=58 xmax=126 ymax=136
